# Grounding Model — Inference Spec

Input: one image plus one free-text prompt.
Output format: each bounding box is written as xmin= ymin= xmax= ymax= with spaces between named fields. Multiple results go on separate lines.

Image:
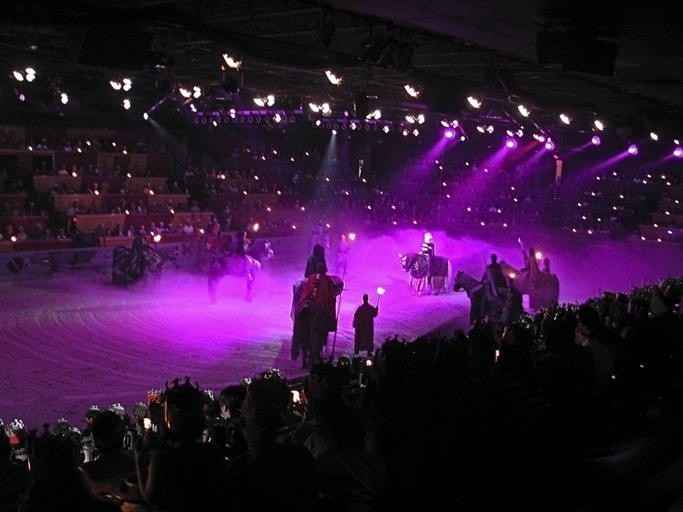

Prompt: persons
xmin=129 ymin=232 xmax=142 ymax=260
xmin=304 ymin=242 xmax=327 ymax=279
xmin=205 ymin=256 xmax=223 ymax=298
xmin=519 ymin=246 xmax=536 ymax=290
xmin=334 ymin=234 xmax=348 ymax=275
xmin=242 ymin=250 xmax=259 ymax=301
xmin=419 ymin=233 xmax=435 ymax=277
xmin=541 ymin=256 xmax=551 ymax=274
xmin=480 ymin=252 xmax=506 ymax=288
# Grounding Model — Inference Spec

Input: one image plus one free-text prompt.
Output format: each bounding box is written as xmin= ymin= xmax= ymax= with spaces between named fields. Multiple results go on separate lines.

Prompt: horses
xmin=316 ymin=234 xmax=333 ymax=263
xmin=109 ymin=230 xmax=276 ymax=303
xmin=498 ymin=258 xmax=561 ymax=307
xmin=453 ymin=270 xmax=524 ymax=327
xmin=293 ymin=274 xmax=335 ymax=371
xmin=397 ymin=251 xmax=454 ymax=297
